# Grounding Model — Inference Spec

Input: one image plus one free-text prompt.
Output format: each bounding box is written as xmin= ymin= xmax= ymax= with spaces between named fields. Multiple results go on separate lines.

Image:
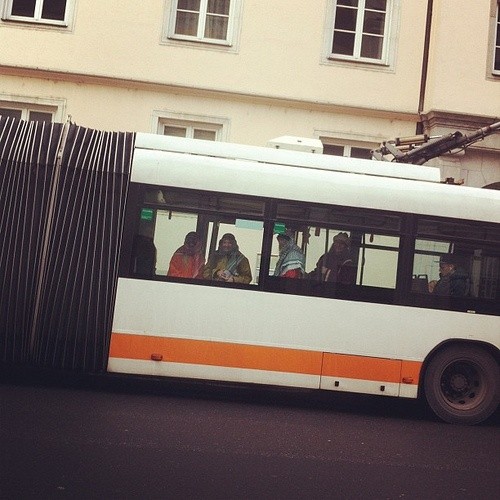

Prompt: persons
xmin=206 ymin=233 xmax=253 ymax=284
xmin=273 ymin=232 xmax=306 ymax=279
xmin=429 ymin=254 xmax=467 ymax=297
xmin=308 ymin=232 xmax=355 ymax=283
xmin=168 ymin=231 xmax=206 ymax=279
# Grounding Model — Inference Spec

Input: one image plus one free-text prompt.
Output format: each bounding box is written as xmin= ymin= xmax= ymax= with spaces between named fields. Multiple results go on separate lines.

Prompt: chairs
xmin=411 ymin=274 xmax=430 ymax=293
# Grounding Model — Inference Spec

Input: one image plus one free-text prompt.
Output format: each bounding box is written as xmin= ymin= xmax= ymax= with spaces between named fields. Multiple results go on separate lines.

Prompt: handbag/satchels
xmin=411 ymin=274 xmax=429 ymax=294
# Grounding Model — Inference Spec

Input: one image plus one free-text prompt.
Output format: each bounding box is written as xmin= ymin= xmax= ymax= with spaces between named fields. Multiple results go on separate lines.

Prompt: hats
xmin=433 ymin=254 xmax=456 ymax=263
xmin=277 ymin=227 xmax=296 ymax=243
xmin=333 ymin=232 xmax=352 ymax=246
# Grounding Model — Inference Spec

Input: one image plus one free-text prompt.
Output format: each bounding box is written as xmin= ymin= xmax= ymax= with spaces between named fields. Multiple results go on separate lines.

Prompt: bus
xmin=0 ymin=114 xmax=500 ymax=426
